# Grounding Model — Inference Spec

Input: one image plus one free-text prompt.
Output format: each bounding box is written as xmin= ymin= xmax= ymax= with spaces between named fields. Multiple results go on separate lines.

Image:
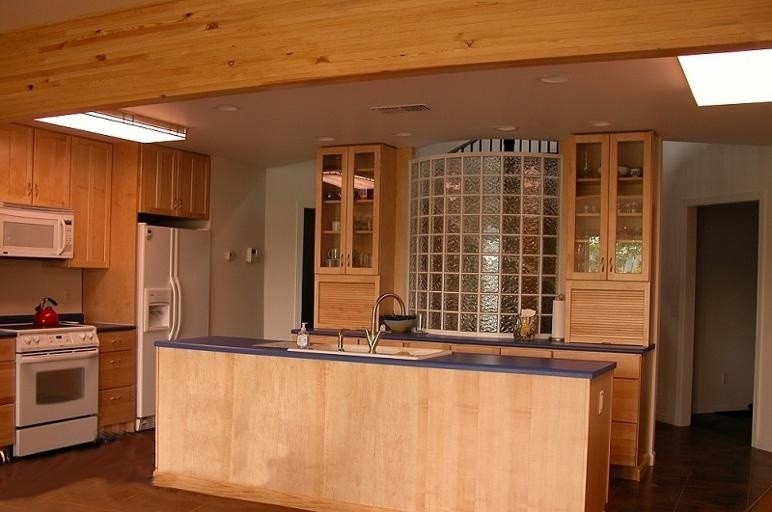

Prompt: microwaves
xmin=1 ymin=203 xmax=75 ymax=260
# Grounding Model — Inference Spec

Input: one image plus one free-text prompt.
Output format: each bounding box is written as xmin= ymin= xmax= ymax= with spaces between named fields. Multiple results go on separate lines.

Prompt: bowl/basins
xmin=324 ymin=190 xmax=358 ymax=200
xmin=381 ymin=314 xmax=417 ymax=333
xmin=578 ymin=165 xmax=641 ymax=178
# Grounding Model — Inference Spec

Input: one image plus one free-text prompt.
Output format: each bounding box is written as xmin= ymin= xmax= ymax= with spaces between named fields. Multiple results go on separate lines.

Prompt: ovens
xmin=15 ymin=348 xmax=99 ymax=460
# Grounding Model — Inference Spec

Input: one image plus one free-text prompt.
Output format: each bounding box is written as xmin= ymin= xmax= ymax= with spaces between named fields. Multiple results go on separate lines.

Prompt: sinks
xmin=287 ymin=343 xmax=453 ymax=361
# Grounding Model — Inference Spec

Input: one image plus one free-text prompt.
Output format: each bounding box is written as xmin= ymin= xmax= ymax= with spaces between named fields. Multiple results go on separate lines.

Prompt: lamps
xmin=322 ymin=171 xmax=374 ymax=190
xmin=36 ymin=109 xmax=188 ymax=143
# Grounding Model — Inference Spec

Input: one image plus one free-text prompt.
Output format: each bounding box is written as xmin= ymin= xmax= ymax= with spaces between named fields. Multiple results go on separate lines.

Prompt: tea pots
xmin=33 ymin=297 xmax=59 ymax=327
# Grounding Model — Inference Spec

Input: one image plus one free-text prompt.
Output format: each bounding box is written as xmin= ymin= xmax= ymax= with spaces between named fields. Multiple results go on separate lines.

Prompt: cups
xmin=323 ymin=247 xmax=373 ymax=269
xmin=332 ymin=214 xmax=372 ymax=231
xmin=576 ymin=226 xmax=641 ymax=240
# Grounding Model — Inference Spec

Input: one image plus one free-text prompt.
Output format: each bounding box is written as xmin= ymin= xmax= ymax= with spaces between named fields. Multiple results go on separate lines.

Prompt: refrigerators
xmin=135 ymin=221 xmax=214 ymax=433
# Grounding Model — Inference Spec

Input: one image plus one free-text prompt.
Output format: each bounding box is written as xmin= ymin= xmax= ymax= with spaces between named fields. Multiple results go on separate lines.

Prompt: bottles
xmin=583 ymin=200 xmax=639 ymax=214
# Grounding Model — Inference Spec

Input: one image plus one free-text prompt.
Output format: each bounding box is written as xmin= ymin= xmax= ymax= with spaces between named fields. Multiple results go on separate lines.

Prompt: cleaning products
xmin=297 ymin=322 xmax=310 ymax=349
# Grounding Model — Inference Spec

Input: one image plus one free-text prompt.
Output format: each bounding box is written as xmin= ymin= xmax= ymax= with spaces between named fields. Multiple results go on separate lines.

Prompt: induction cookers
xmin=1 ymin=321 xmax=96 ymax=334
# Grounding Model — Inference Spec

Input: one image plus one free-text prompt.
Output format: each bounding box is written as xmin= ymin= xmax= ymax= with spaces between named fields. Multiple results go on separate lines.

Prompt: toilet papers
xmin=552 ymin=299 xmax=566 ymax=338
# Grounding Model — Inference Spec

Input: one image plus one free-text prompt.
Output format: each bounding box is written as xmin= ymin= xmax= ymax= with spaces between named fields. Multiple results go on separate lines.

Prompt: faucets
xmin=365 ymin=293 xmax=406 ymax=354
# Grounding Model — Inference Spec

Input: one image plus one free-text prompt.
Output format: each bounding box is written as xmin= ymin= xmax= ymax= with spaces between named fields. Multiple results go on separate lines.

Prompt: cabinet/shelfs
xmin=564 ymin=128 xmax=659 ymax=347
xmin=138 ymin=141 xmax=210 ymax=220
xmin=313 ymin=142 xmax=397 ymax=329
xmin=0 ymin=122 xmax=71 ymax=210
xmin=552 ymin=349 xmax=648 ymax=466
xmin=98 ymin=329 xmax=136 ymax=424
xmin=68 ymin=135 xmax=113 ymax=269
xmin=0 ymin=337 xmax=16 ymax=448
